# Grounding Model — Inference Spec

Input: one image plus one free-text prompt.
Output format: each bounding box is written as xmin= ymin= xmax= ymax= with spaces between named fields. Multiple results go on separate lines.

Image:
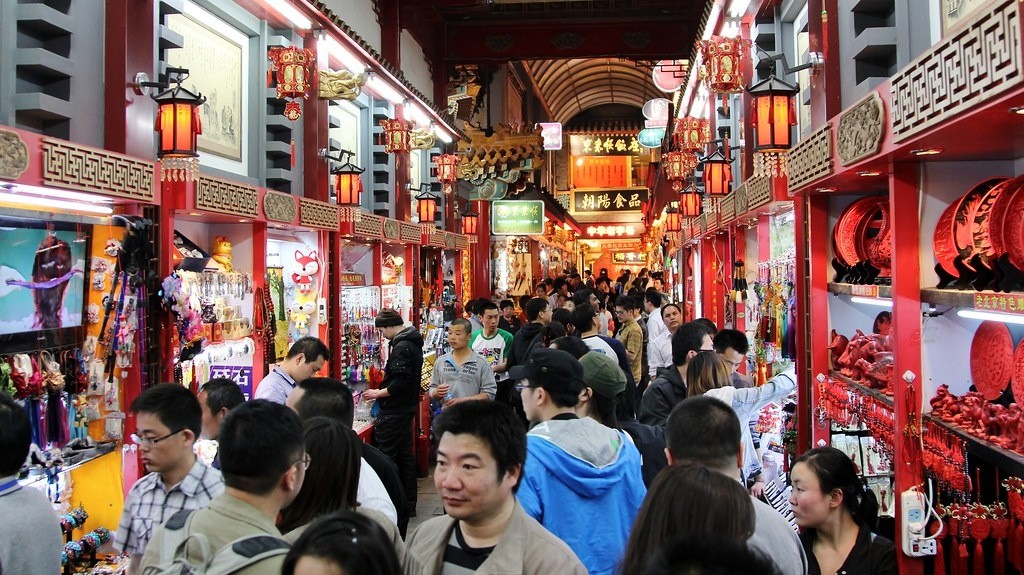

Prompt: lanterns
xmin=149 ymin=86 xmax=207 ymax=182
xmin=461 ymin=211 xmax=479 ymax=235
xmin=432 ymin=154 xmax=459 ymax=219
xmin=379 ymin=117 xmax=415 ymax=203
xmin=666 ymin=36 xmax=752 ymax=205
xmin=416 ymin=191 xmax=439 ymax=235
xmin=665 ymin=151 xmax=735 ymax=232
xmin=268 ymin=46 xmax=318 ymax=169
xmin=331 ymin=163 xmax=366 ymax=222
xmin=744 ymin=75 xmax=800 ymax=153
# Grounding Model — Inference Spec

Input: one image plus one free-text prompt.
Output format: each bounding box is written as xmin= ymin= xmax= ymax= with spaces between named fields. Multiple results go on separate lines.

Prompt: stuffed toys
xmin=290 ymin=289 xmax=317 ymax=328
xmin=291 ymin=250 xmax=319 ymax=292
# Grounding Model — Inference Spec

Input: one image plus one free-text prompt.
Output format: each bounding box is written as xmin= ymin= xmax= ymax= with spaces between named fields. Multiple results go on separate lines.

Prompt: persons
xmin=402 ymin=400 xmax=590 ymax=575
xmin=0 ymin=391 xmax=62 ymax=575
xmin=790 ymin=446 xmax=900 ymax=575
xmin=141 ymin=398 xmax=305 ymax=575
xmin=510 ymin=347 xmax=648 ymax=575
xmin=282 ymin=512 xmax=404 ymax=575
xmin=621 ymin=462 xmax=785 ymax=575
xmin=664 ymin=396 xmax=808 ymax=575
xmin=196 ymin=267 xmax=799 ymax=534
xmin=113 ymin=382 xmax=229 ymax=575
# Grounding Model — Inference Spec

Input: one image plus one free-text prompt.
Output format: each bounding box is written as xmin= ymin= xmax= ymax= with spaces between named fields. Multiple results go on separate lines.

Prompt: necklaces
xmin=252 ymin=287 xmax=268 ymax=342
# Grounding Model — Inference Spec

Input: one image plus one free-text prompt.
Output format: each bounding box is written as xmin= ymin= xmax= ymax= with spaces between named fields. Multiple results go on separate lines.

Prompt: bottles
xmin=763 ymin=454 xmax=777 ymax=485
xmin=442 ymin=384 xmax=453 ymax=405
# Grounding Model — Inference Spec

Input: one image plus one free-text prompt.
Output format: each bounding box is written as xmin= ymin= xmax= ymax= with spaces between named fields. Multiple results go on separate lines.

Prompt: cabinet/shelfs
xmin=812 ymin=90 xmax=1024 ymax=466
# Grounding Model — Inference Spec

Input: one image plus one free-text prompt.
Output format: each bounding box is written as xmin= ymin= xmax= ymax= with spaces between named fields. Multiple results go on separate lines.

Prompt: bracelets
xmin=60 ymin=509 xmax=110 ymax=564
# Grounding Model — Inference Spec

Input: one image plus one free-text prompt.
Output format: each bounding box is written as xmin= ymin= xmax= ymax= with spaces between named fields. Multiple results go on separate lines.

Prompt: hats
xmin=577 ymin=351 xmax=628 ymax=405
xmin=509 ymin=348 xmax=584 ymax=397
xmin=375 ymin=307 xmax=404 ymax=327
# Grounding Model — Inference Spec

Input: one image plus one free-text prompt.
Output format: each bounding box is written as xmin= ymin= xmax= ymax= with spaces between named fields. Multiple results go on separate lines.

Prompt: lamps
xmin=135 ymin=64 xmax=206 ymax=158
xmin=744 ymin=52 xmax=821 ymax=151
xmin=698 ymin=138 xmax=739 ymax=198
xmin=406 ymin=182 xmax=439 ymax=223
xmin=322 ymin=148 xmax=366 ymax=207
xmin=455 ymin=201 xmax=479 ymax=234
xmin=679 ymin=182 xmax=707 ymax=217
xmin=667 ymin=202 xmax=682 ymax=231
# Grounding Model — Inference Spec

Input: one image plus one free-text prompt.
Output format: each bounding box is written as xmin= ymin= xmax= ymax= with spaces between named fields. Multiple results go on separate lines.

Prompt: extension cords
xmin=900 ymin=491 xmax=937 ymax=557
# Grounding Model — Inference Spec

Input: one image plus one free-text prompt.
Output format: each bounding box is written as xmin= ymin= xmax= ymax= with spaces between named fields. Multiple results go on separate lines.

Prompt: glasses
xmin=688 ymin=347 xmax=718 ymax=355
xmin=512 ymin=380 xmax=545 ymax=389
xmin=284 ymin=449 xmax=312 ymax=477
xmin=613 ymin=309 xmax=627 ymax=314
xmin=131 ymin=426 xmax=193 ymax=447
